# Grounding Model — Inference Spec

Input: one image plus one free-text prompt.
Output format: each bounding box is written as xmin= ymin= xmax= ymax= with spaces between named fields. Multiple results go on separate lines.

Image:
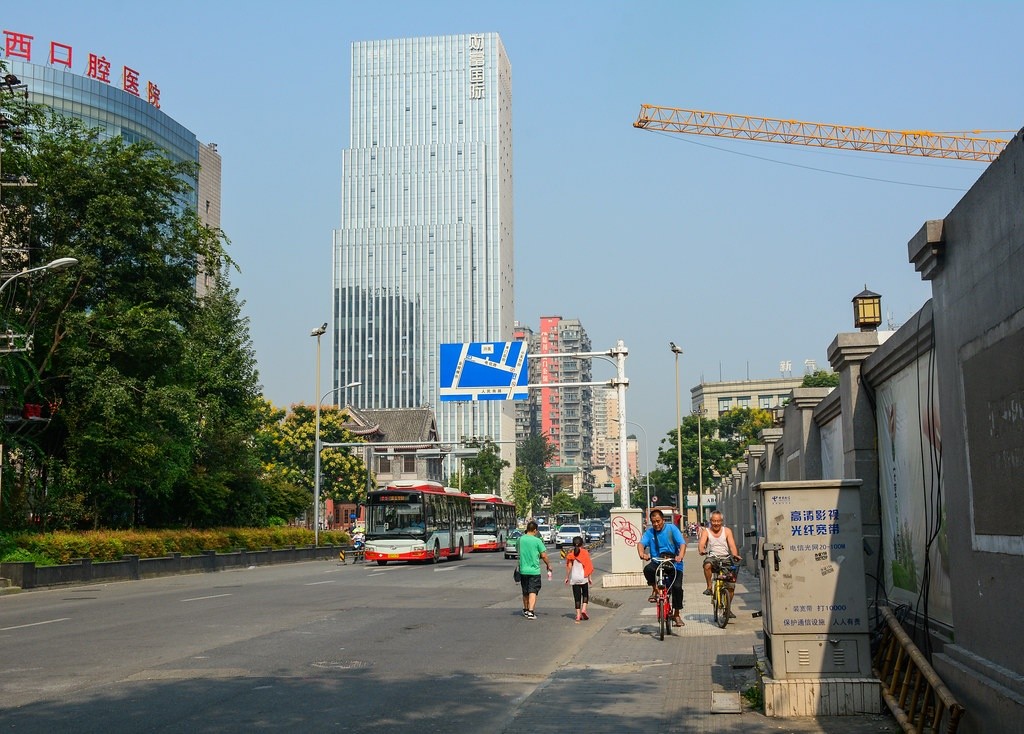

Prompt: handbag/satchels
xmin=659 ymin=552 xmax=676 ymax=566
xmin=514 ymin=565 xmax=521 ymax=582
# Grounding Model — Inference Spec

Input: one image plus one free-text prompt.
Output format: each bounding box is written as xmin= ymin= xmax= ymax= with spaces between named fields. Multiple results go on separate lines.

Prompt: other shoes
xmin=527 ymin=612 xmax=536 ymax=619
xmin=582 ymin=610 xmax=590 ymax=620
xmin=703 ymin=589 xmax=713 ymax=594
xmin=575 ymin=615 xmax=582 ymax=621
xmin=522 ymin=608 xmax=528 ymax=617
xmin=724 ymin=610 xmax=737 ymax=619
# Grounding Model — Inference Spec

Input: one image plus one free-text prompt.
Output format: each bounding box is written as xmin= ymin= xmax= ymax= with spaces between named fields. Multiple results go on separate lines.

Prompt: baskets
xmin=714 ymin=562 xmax=740 ymax=581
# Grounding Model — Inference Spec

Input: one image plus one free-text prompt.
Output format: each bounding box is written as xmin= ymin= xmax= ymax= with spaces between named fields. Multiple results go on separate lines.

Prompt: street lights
xmin=690 ymin=409 xmax=708 ymax=525
xmin=314 ymin=381 xmax=363 ymax=547
xmin=612 ymin=418 xmax=651 ymax=527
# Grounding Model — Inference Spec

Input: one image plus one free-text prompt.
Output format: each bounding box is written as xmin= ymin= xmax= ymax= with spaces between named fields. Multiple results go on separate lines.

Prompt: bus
xmin=469 ymin=493 xmax=517 ymax=552
xmin=362 ymin=480 xmax=474 ymax=566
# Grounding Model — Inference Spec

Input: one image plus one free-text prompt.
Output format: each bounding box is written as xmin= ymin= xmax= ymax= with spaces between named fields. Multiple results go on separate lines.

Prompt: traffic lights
xmin=604 ymin=483 xmax=615 ymax=487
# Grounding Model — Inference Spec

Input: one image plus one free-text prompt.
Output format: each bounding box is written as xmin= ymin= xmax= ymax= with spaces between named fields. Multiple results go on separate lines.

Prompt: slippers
xmin=648 ymin=594 xmax=658 ymax=602
xmin=673 ymin=616 xmax=684 ymax=626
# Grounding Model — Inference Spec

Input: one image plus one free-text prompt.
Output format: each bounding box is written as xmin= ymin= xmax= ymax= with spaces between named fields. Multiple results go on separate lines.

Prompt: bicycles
xmin=700 ymin=550 xmax=743 ymax=628
xmin=643 ymin=557 xmax=683 ymax=641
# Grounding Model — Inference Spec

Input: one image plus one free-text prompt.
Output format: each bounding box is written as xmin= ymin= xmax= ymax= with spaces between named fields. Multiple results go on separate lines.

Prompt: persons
xmin=410 ymin=513 xmax=425 ymax=530
xmin=564 ymin=536 xmax=594 ymax=622
xmin=698 ymin=510 xmax=743 ymax=618
xmin=327 ymin=511 xmax=334 ymax=530
xmin=515 ymin=521 xmax=553 ymax=620
xmin=352 ymin=529 xmax=365 ymax=564
xmin=637 ymin=509 xmax=687 ymax=626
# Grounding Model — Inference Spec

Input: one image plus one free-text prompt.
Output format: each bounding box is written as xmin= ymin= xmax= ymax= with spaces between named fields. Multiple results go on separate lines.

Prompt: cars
xmin=555 ymin=524 xmax=586 ymax=549
xmin=578 ymin=518 xmax=611 ymax=543
xmin=538 ymin=524 xmax=555 ymax=544
xmin=503 ymin=529 xmax=545 ymax=559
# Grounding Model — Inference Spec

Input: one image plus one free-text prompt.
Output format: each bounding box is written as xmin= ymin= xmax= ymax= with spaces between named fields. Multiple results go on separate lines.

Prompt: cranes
xmin=631 ymin=103 xmax=1019 ymax=163
